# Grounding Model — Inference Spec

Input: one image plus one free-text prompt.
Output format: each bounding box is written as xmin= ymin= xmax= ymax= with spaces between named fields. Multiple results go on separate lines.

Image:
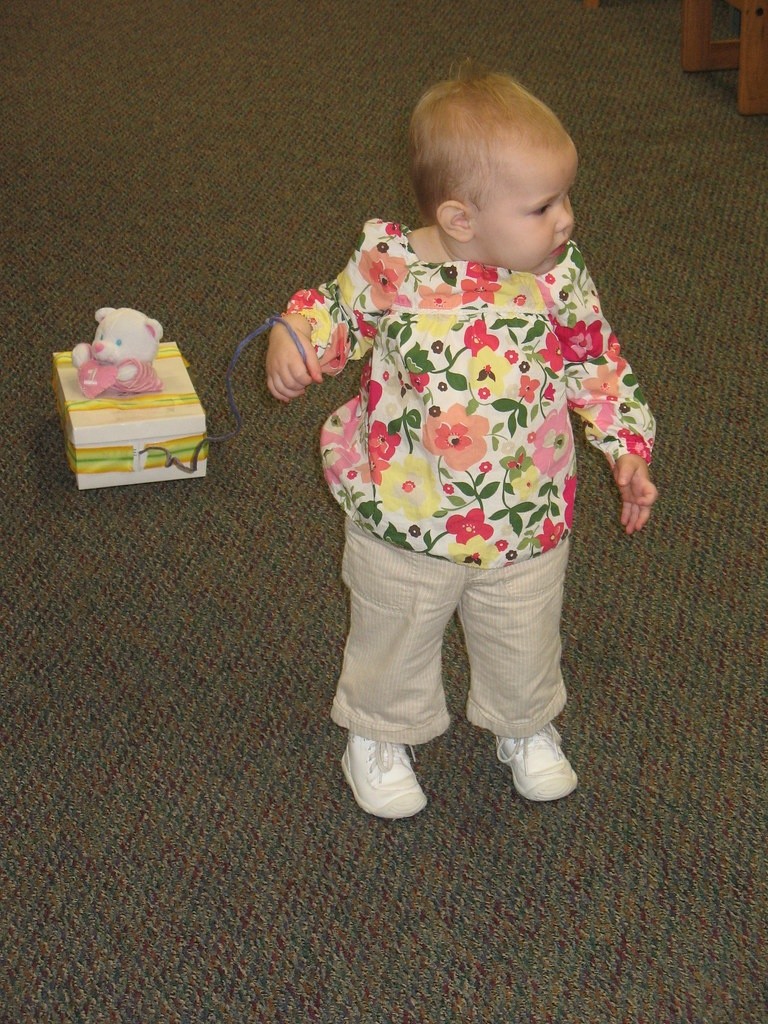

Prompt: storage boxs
xmin=52 ymin=341 xmax=207 ymax=491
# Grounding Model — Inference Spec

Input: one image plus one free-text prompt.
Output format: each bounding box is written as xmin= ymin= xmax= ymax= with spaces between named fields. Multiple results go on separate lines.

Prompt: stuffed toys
xmin=72 ymin=306 xmax=163 ymax=399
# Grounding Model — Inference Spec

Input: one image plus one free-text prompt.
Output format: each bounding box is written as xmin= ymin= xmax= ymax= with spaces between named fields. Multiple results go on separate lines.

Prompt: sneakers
xmin=341 ymin=730 xmax=428 ymax=818
xmin=496 ymin=721 xmax=578 ymax=801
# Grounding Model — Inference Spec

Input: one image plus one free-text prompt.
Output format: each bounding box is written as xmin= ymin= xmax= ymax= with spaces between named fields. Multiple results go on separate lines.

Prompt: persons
xmin=267 ymin=71 xmax=656 ymax=822
